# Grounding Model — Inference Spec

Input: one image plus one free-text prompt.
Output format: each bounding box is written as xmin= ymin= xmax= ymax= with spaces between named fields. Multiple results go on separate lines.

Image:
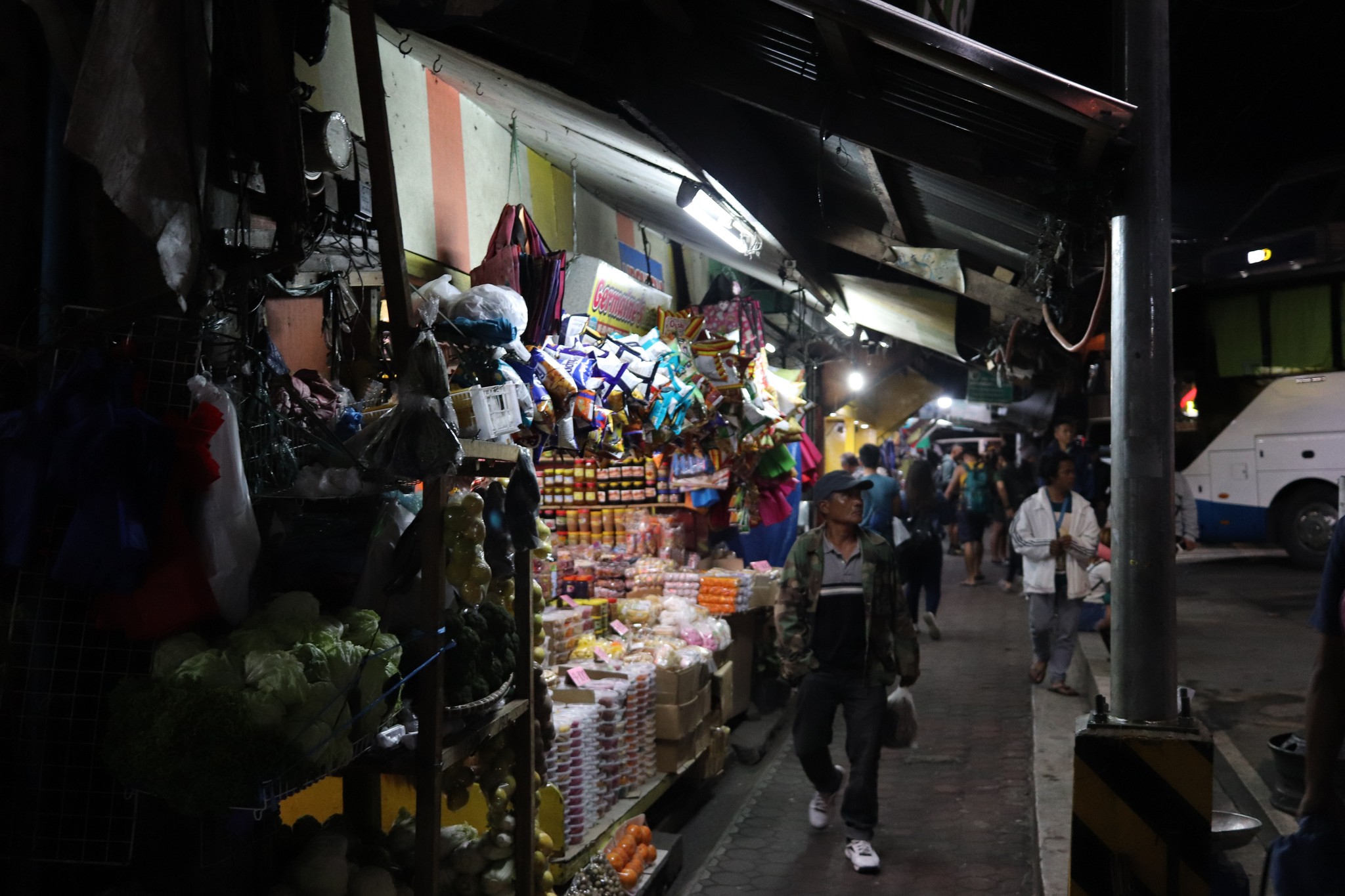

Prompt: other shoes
xmin=1045 ymin=680 xmax=1081 ymax=698
xmin=996 ymin=580 xmax=1017 ymax=594
xmin=923 ymin=611 xmax=942 ymax=641
xmin=913 ymin=627 xmax=922 ymax=633
xmin=1027 ymin=658 xmax=1047 ymax=684
xmin=963 ymin=574 xmax=982 ymax=588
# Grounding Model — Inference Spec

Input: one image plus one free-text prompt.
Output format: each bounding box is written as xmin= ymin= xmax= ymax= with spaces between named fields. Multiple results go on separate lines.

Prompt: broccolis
xmin=384 ymin=602 xmax=521 ymax=711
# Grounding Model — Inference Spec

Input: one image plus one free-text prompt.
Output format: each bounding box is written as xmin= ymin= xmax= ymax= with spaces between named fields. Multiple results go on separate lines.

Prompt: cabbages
xmin=152 ymin=587 xmax=401 ymax=771
xmin=275 ymin=805 xmax=522 ymax=896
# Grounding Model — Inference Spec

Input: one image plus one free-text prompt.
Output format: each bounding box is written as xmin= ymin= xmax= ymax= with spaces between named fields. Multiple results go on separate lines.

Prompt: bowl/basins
xmin=545 ymin=661 xmax=657 ymax=851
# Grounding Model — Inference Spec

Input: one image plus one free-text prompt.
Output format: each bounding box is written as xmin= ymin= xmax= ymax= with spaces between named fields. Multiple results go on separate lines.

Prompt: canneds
xmin=536 ymin=457 xmax=679 ymax=545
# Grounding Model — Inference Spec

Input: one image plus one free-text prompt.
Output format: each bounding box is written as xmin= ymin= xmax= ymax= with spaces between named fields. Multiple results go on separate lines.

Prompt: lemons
xmin=443 ymin=734 xmax=556 ymax=896
xmin=444 ymin=492 xmax=491 ymax=606
xmin=488 ymin=575 xmax=548 ymax=665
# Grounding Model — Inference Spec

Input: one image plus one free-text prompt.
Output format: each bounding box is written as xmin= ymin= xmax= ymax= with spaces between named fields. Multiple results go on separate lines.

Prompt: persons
xmin=777 ymin=469 xmax=919 ymax=873
xmin=1009 ymin=455 xmax=1100 ymax=696
xmin=942 ymin=418 xmax=1199 ymax=632
xmin=839 ymin=444 xmax=944 ymax=641
xmin=1297 ymin=514 xmax=1345 ymax=896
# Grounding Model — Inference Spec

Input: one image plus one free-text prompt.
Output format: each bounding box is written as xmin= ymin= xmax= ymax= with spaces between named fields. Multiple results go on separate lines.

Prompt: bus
xmin=1082 ymin=219 xmax=1345 ymax=572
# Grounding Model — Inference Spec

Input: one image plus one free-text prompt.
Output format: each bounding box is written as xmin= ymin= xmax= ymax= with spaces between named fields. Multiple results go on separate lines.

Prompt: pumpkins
xmin=607 ymin=823 xmax=656 ymax=889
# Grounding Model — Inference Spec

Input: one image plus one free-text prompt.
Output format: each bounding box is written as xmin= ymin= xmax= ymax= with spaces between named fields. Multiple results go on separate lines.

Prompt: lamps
xmin=822 ymin=302 xmax=857 ymax=337
xmin=675 ymin=174 xmax=762 ymax=258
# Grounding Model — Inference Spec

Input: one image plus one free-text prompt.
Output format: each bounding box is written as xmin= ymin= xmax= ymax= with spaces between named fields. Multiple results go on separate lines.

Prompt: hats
xmin=814 ymin=471 xmax=876 ymax=505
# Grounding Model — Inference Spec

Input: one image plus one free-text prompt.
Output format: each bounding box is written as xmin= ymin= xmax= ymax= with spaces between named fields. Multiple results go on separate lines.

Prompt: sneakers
xmin=844 ymin=838 xmax=883 ymax=874
xmin=807 ymin=764 xmax=846 ymax=831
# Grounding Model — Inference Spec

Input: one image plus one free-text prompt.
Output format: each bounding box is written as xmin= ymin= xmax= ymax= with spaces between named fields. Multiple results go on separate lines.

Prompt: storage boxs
xmin=357 ymin=380 xmax=522 ymax=441
xmin=655 ymin=661 xmax=733 ymax=772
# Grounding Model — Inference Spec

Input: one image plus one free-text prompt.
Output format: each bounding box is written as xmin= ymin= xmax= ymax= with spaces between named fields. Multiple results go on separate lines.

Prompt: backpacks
xmin=958 ymin=458 xmax=996 ymax=516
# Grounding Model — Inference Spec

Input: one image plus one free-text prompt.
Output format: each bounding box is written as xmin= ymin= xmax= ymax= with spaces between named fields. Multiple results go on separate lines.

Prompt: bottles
xmin=561 ymin=574 xmax=617 ymax=640
xmin=534 ymin=457 xmax=678 ymax=552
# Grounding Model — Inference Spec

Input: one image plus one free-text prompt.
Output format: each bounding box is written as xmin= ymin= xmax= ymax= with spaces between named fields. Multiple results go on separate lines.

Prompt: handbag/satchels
xmin=464 ymin=203 xmax=570 ymax=347
xmin=884 ymin=682 xmax=918 ymax=750
xmin=893 ymin=517 xmax=910 ymax=547
xmin=688 ymin=271 xmax=767 ymax=359
